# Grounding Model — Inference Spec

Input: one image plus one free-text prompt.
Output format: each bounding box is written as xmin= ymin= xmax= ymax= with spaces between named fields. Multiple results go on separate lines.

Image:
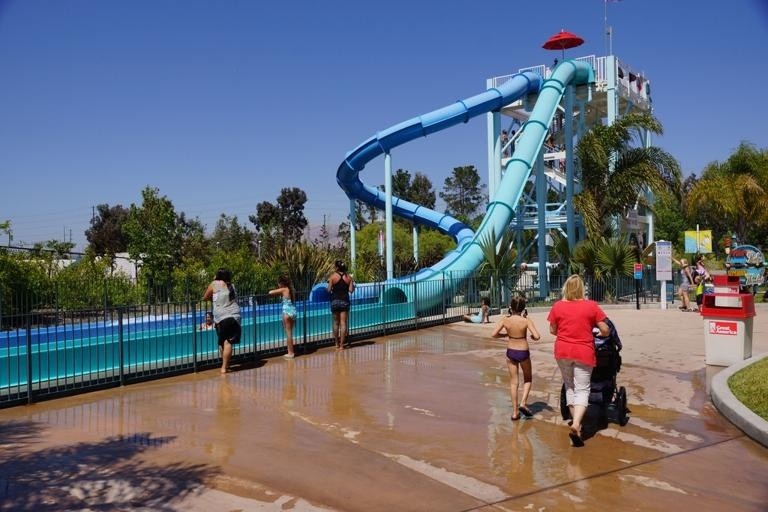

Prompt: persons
xmin=558 ymin=144 xmax=566 ymax=168
xmin=463 ymin=297 xmax=491 ymax=323
xmin=502 ymin=129 xmax=516 ymax=158
xmin=547 ymin=274 xmax=610 ymax=447
xmin=200 ymin=312 xmax=215 ymax=330
xmin=203 ymin=268 xmax=241 ymax=373
xmin=670 ymin=255 xmax=710 ymax=312
xmin=327 ymin=259 xmax=354 ymax=350
xmin=491 ymin=296 xmax=540 ymax=420
xmin=269 ymin=275 xmax=297 ymax=358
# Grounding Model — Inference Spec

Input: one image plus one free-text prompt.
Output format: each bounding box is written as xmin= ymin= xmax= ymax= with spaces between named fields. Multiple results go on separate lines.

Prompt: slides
xmin=1 ymin=68 xmax=542 ymax=347
xmin=0 ymin=59 xmax=594 ymax=390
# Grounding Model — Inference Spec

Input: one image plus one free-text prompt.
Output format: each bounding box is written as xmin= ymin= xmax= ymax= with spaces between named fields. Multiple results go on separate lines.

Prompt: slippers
xmin=568 ymin=420 xmax=584 ymax=447
xmin=511 ymin=404 xmax=533 ymax=421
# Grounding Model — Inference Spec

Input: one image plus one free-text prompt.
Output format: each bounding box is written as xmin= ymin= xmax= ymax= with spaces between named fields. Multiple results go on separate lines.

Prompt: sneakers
xmin=678 ymin=305 xmax=693 ymax=312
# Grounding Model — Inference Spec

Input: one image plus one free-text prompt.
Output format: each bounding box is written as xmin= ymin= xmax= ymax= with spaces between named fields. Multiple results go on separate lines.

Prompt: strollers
xmin=557 ymin=317 xmax=631 ymax=425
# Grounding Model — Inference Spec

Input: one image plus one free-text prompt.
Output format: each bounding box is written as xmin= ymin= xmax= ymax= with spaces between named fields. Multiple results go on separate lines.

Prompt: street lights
xmin=258 ymin=240 xmax=261 ymax=259
xmin=215 ymin=240 xmax=221 ymax=254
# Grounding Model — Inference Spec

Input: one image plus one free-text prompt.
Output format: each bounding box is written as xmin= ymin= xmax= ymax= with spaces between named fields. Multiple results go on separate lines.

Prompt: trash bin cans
xmin=714 ymin=274 xmax=740 ymax=293
xmin=701 ymin=293 xmax=757 ymax=367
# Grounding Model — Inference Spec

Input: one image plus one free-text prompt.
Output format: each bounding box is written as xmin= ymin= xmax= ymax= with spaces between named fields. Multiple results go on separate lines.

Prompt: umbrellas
xmin=542 ymin=28 xmax=584 ymax=60
